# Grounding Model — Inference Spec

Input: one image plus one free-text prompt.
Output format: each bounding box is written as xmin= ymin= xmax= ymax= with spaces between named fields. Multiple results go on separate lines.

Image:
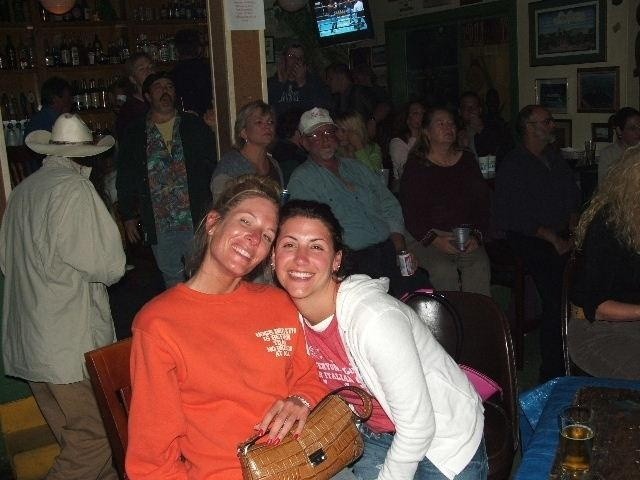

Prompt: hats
xmin=299 ymin=107 xmax=335 ymax=134
xmin=25 ymin=113 xmax=115 ymax=157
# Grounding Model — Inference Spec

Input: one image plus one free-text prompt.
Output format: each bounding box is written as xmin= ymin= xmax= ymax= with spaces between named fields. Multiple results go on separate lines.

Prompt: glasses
xmin=528 ymin=117 xmax=554 ymax=126
xmin=306 ymin=130 xmax=333 ymax=138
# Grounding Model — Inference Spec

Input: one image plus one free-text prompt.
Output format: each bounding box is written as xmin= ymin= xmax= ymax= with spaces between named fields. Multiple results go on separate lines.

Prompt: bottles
xmin=136 ymin=220 xmax=145 ymax=247
xmin=366 ymin=117 xmax=377 ymax=138
xmin=0 ymin=0 xmax=211 ymax=147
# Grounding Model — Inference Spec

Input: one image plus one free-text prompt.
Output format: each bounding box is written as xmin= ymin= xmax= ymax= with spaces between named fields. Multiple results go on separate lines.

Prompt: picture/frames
xmin=265 ymin=36 xmax=275 ymax=64
xmin=535 ymin=78 xmax=568 ymax=114
xmin=553 ymin=119 xmax=572 ymax=148
xmin=371 ymin=45 xmax=387 ymax=67
xmin=577 ymin=66 xmax=620 ymax=113
xmin=591 ymin=123 xmax=613 ymax=142
xmin=528 ymin=0 xmax=607 ymax=67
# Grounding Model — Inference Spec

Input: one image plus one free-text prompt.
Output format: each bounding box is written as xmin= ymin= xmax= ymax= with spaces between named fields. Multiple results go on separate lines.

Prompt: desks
xmin=513 ymin=376 xmax=639 ymax=480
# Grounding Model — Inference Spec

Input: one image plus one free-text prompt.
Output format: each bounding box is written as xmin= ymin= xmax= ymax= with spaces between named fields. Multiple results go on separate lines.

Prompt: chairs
xmin=84 ymin=336 xmax=132 ymax=480
xmin=562 ymin=242 xmax=640 ymax=380
xmin=400 ymin=292 xmax=520 ymax=480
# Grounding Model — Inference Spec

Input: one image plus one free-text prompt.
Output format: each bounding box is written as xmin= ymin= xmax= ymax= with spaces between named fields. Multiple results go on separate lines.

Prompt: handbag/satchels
xmin=237 ymin=394 xmax=366 ymax=480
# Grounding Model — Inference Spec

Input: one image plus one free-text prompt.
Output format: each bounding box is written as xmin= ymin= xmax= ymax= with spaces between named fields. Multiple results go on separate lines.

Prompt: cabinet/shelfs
xmin=0 ymin=0 xmax=214 ymax=144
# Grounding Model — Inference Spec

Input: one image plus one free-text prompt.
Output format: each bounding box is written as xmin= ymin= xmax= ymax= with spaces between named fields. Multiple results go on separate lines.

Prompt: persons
xmin=0 ymin=112 xmax=127 ymax=480
xmin=598 ymin=107 xmax=640 ymax=192
xmin=490 ymin=104 xmax=595 ymax=385
xmin=272 ymin=200 xmax=489 ymax=480
xmin=330 ymin=0 xmax=366 ymax=33
xmin=572 ymin=145 xmax=640 ymax=323
xmin=25 ymin=44 xmax=595 ymax=299
xmin=124 ymin=176 xmax=358 ymax=480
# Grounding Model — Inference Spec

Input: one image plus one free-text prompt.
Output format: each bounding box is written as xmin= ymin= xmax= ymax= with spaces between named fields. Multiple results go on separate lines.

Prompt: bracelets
xmin=286 ymin=395 xmax=310 ymax=409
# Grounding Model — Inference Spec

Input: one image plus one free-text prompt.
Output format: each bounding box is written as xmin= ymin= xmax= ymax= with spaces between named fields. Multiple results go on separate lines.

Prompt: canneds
xmin=454 ymin=227 xmax=471 ymax=252
xmin=397 ymin=250 xmax=414 ymax=276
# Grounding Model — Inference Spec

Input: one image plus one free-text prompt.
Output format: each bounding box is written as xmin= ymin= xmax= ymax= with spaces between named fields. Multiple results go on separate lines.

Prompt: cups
xmin=453 ymin=228 xmax=469 ymax=252
xmin=479 ymin=157 xmax=489 ymax=174
xmin=584 ymin=136 xmax=597 ymax=160
xmin=558 ymin=405 xmax=598 ymax=476
xmin=487 ymin=156 xmax=496 ymax=172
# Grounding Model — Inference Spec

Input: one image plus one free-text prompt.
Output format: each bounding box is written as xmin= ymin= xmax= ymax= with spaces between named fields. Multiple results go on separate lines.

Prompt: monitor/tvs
xmin=307 ymin=0 xmax=375 ymax=47
